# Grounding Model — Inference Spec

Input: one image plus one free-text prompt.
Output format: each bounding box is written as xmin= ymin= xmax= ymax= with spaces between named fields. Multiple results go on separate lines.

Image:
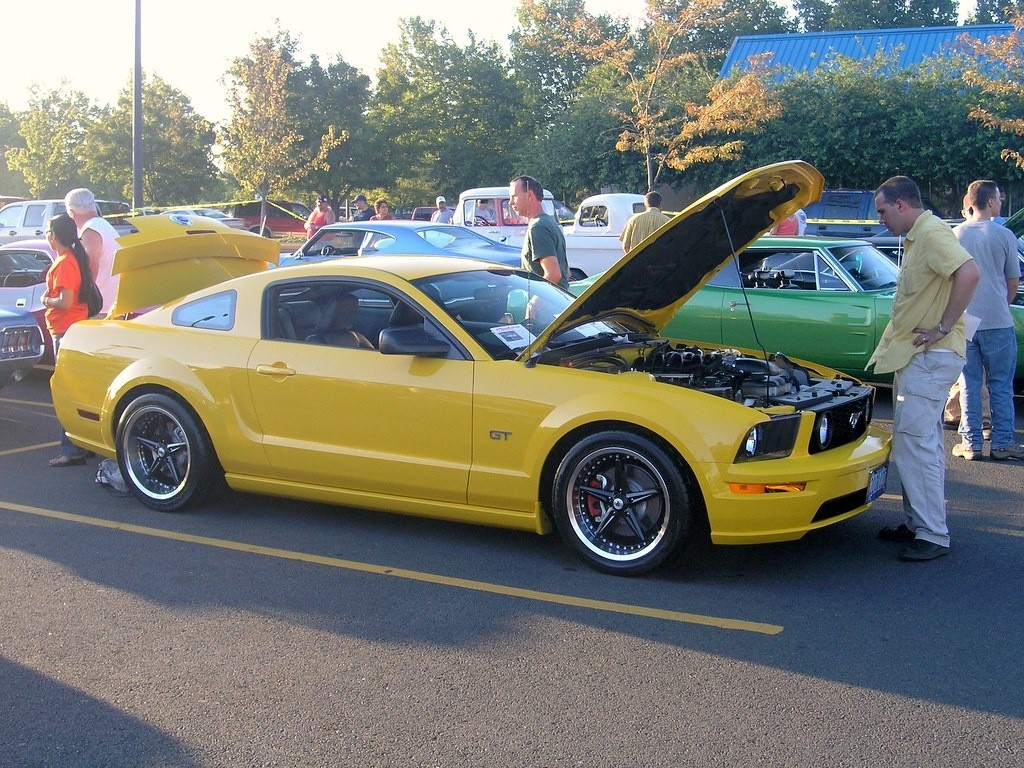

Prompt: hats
xmin=351 ymin=195 xmax=366 ymax=202
xmin=436 ymin=196 xmax=446 ymax=204
xmin=315 ymin=195 xmax=328 ymax=202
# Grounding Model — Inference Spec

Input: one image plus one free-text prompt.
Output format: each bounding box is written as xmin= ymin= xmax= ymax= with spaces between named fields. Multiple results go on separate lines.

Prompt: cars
xmin=1 ymin=185 xmax=1024 ymax=306
xmin=0 ymin=303 xmax=47 ymax=393
xmin=507 ymin=234 xmax=1024 ymax=412
xmin=278 ymin=220 xmax=522 ymax=321
xmin=0 ymin=237 xmax=163 ymax=360
xmin=56 ymin=159 xmax=893 ymax=577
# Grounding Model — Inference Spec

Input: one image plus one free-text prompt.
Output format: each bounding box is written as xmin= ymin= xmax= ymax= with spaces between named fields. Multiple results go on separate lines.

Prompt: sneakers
xmin=952 ymin=444 xmax=982 ymax=461
xmin=991 ymin=443 xmax=1024 ymax=462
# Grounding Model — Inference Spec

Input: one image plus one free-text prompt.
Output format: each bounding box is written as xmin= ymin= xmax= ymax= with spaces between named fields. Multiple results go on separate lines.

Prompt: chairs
xmin=280 ymin=286 xmax=449 ymax=347
xmin=748 ymin=269 xmax=800 ymax=289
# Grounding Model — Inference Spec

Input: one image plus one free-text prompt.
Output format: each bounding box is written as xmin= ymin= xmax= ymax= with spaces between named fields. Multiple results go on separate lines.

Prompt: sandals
xmin=50 ymin=454 xmax=87 ymax=467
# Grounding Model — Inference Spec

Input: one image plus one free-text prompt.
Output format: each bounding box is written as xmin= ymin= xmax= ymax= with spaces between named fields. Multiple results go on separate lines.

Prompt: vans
xmin=0 ymin=199 xmax=132 ymax=247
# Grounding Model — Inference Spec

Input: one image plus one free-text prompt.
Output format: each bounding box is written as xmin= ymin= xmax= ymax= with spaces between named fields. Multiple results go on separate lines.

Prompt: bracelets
xmin=936 ymin=321 xmax=954 ymax=335
xmin=527 ymin=301 xmax=539 ymax=312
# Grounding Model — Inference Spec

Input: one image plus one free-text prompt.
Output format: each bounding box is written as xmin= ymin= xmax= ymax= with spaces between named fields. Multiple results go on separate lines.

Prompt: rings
xmin=923 ymin=337 xmax=927 ymax=341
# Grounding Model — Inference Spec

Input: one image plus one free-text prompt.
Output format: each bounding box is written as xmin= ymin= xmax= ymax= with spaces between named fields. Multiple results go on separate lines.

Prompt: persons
xmin=369 ymin=197 xmax=395 ymax=242
xmin=474 ymin=200 xmax=493 ymax=222
xmin=940 ymin=177 xmax=1024 ymax=464
xmin=771 ymin=206 xmax=809 ymax=237
xmin=348 ymin=194 xmax=375 ymax=247
xmin=508 ymin=175 xmax=571 ymax=326
xmin=38 ymin=213 xmax=99 ymax=463
xmin=62 ymin=187 xmax=123 ymax=315
xmin=430 ymin=195 xmax=455 ymax=225
xmin=619 ymin=190 xmax=673 ymax=254
xmin=862 ymin=176 xmax=979 ymax=560
xmin=302 ymin=193 xmax=336 ymax=249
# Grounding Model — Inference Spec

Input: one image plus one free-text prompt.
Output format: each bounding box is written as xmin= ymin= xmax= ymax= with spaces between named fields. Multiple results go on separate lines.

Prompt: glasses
xmin=964 ymin=207 xmax=973 ymax=214
xmin=46 ymin=230 xmax=55 ymax=235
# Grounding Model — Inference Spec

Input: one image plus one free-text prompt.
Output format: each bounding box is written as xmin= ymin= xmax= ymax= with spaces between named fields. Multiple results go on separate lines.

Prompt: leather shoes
xmin=900 ymin=539 xmax=950 ymax=559
xmin=879 ymin=524 xmax=916 ymax=539
